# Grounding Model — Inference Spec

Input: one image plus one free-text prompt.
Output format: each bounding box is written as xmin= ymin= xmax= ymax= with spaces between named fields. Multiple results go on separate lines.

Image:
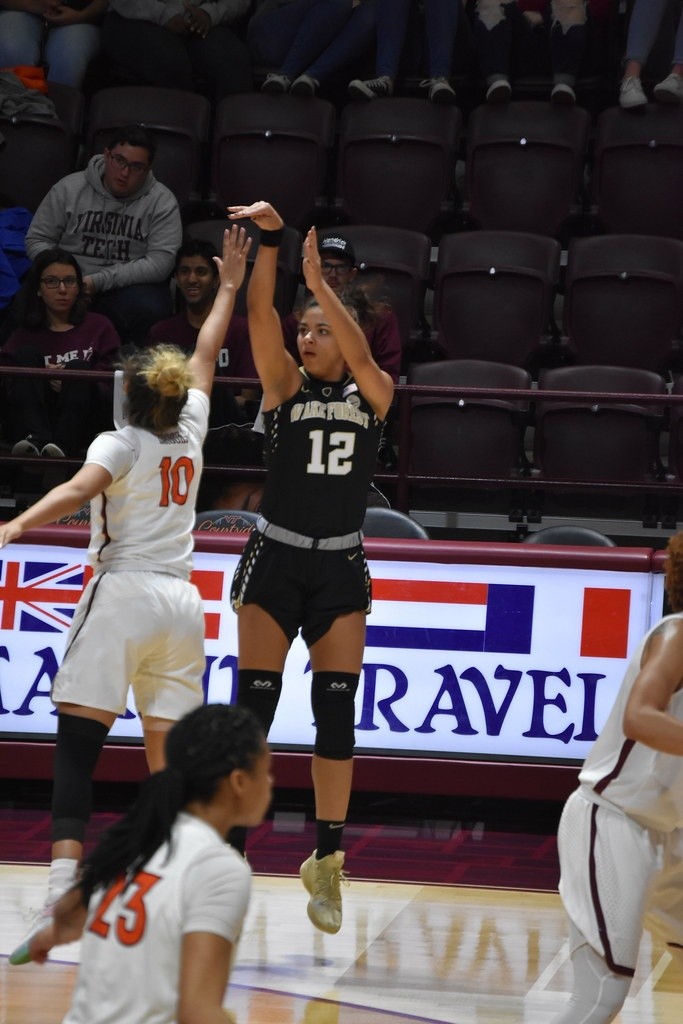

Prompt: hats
xmin=318 ymin=234 xmax=355 ymax=262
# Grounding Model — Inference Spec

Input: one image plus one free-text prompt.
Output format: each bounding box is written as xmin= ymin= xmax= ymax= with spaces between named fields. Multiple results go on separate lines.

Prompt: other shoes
xmin=485 ymin=75 xmax=512 ymax=103
xmin=551 ymin=72 xmax=579 ymax=104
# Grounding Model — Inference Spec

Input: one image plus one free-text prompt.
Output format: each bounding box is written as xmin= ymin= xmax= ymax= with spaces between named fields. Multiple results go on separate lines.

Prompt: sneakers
xmin=263 ymin=74 xmax=288 ymax=94
xmin=287 ymin=75 xmax=317 ymax=96
xmin=653 ymin=74 xmax=682 ymax=101
xmin=350 ymin=75 xmax=394 ymax=101
xmin=429 ymin=77 xmax=456 ymax=104
xmin=300 ymin=849 xmax=350 ymax=935
xmin=617 ymin=77 xmax=648 ymax=108
xmin=9 ymin=901 xmax=57 ymax=965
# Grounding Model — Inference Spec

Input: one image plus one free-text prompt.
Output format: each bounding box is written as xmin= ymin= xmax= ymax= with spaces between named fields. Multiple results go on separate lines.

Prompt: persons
xmin=0 ymin=127 xmax=402 ymax=514
xmin=0 ymin=226 xmax=253 ymax=964
xmin=521 ymin=531 xmax=683 ymax=1024
xmin=29 ymin=703 xmax=272 ymax=1024
xmin=221 ymin=201 xmax=395 ymax=935
xmin=0 ymin=0 xmax=683 ymax=109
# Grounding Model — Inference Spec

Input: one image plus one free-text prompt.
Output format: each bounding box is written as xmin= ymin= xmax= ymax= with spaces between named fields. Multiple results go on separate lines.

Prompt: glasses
xmin=321 ymin=263 xmax=352 ymax=275
xmin=41 ymin=277 xmax=79 ymax=288
xmin=109 ymin=150 xmax=152 ymax=176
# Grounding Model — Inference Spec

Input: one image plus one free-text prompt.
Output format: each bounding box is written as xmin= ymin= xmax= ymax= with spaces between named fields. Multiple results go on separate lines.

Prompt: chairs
xmin=0 ymin=87 xmax=683 ymax=552
